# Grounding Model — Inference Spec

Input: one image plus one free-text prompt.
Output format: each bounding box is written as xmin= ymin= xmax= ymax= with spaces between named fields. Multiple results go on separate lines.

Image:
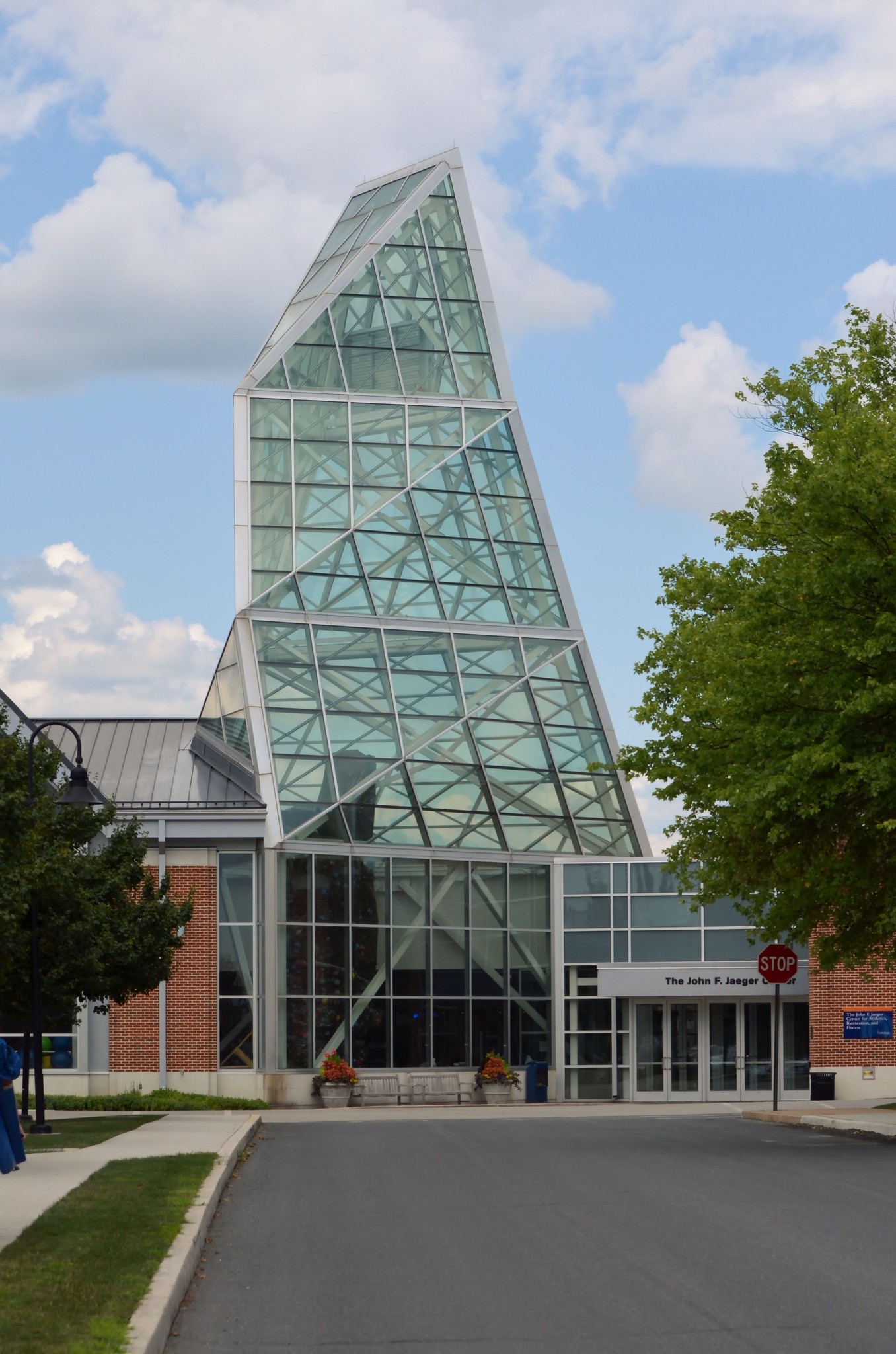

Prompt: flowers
xmin=310 ymin=1048 xmax=358 ymax=1096
xmin=474 ymin=1049 xmax=522 ymax=1092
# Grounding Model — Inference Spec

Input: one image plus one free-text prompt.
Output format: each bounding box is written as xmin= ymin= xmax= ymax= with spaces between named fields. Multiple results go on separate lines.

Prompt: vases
xmin=481 ymin=1079 xmax=512 ymax=1104
xmin=320 ymin=1082 xmax=351 ymax=1108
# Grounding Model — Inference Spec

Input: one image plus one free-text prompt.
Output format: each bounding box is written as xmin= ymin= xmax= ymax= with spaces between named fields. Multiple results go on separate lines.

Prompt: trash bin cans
xmin=525 ymin=1061 xmax=550 ymax=1102
xmin=807 ymin=1071 xmax=835 ymax=1101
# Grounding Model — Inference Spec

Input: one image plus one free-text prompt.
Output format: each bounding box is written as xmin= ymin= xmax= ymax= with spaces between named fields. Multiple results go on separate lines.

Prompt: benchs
xmin=350 ymin=1074 xmax=415 ymax=1107
xmin=407 ymin=1072 xmax=475 ymax=1105
